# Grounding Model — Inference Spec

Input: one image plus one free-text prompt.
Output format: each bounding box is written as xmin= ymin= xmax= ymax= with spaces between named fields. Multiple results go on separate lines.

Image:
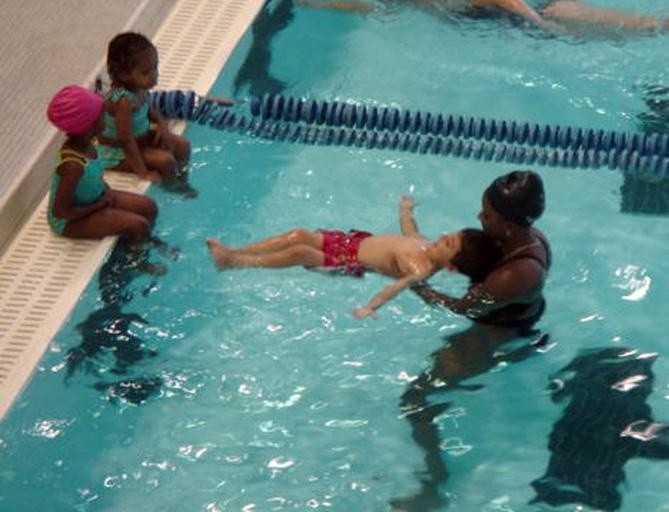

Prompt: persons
xmin=206 ymin=193 xmax=503 ymax=317
xmin=46 ymin=84 xmax=177 ymax=274
xmin=296 ymin=0 xmax=668 ymax=39
xmin=92 ymin=33 xmax=199 ymax=198
xmin=389 ymin=170 xmax=553 ymax=512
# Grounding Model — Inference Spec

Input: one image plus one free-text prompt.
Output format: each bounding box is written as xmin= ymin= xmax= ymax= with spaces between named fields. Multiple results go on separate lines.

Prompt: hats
xmin=485 ymin=171 xmax=546 ymax=229
xmin=46 ymin=85 xmax=104 ymax=135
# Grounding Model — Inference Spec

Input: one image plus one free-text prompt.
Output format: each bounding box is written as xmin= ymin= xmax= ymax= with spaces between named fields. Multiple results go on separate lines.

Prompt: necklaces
xmin=504 ymin=236 xmax=541 ymax=260
